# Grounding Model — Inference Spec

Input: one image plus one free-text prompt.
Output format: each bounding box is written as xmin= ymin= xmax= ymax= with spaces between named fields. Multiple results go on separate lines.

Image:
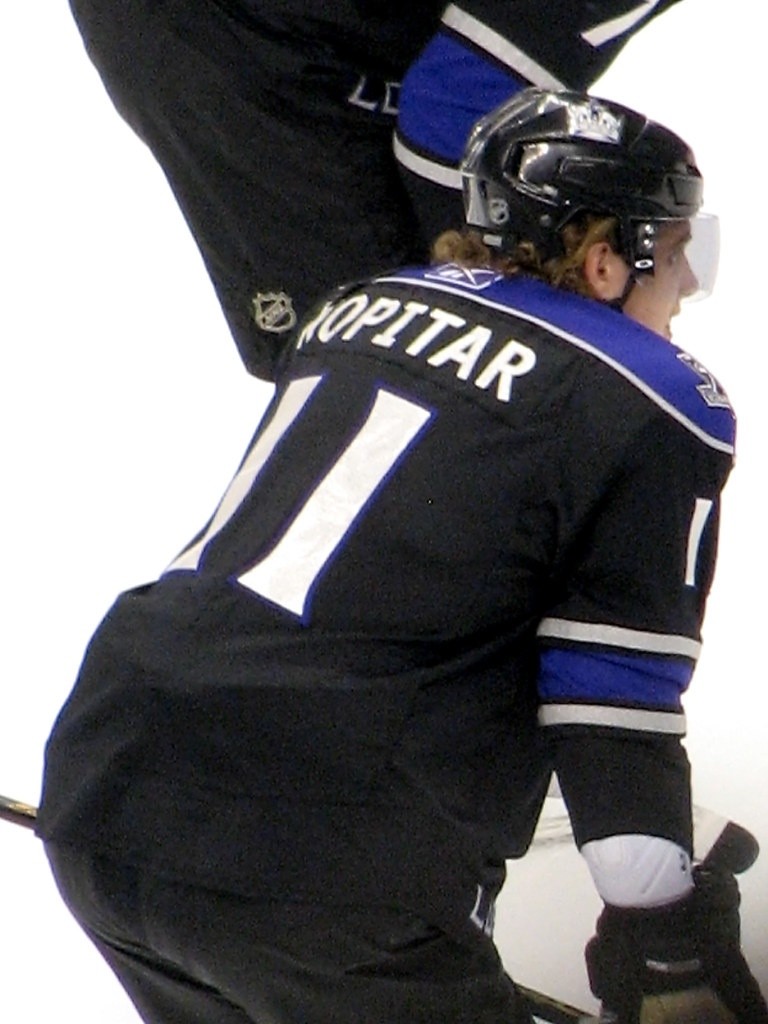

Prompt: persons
xmin=37 ymin=88 xmax=768 ymax=1024
xmin=69 ymin=0 xmax=680 ymax=384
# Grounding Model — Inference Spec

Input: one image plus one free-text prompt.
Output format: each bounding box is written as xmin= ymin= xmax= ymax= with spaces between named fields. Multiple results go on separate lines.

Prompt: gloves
xmin=583 ymin=818 xmax=768 ymax=1024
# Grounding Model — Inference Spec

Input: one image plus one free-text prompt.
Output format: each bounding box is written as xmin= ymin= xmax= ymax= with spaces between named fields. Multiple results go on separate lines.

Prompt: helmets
xmin=456 ymin=86 xmax=718 ymax=296
